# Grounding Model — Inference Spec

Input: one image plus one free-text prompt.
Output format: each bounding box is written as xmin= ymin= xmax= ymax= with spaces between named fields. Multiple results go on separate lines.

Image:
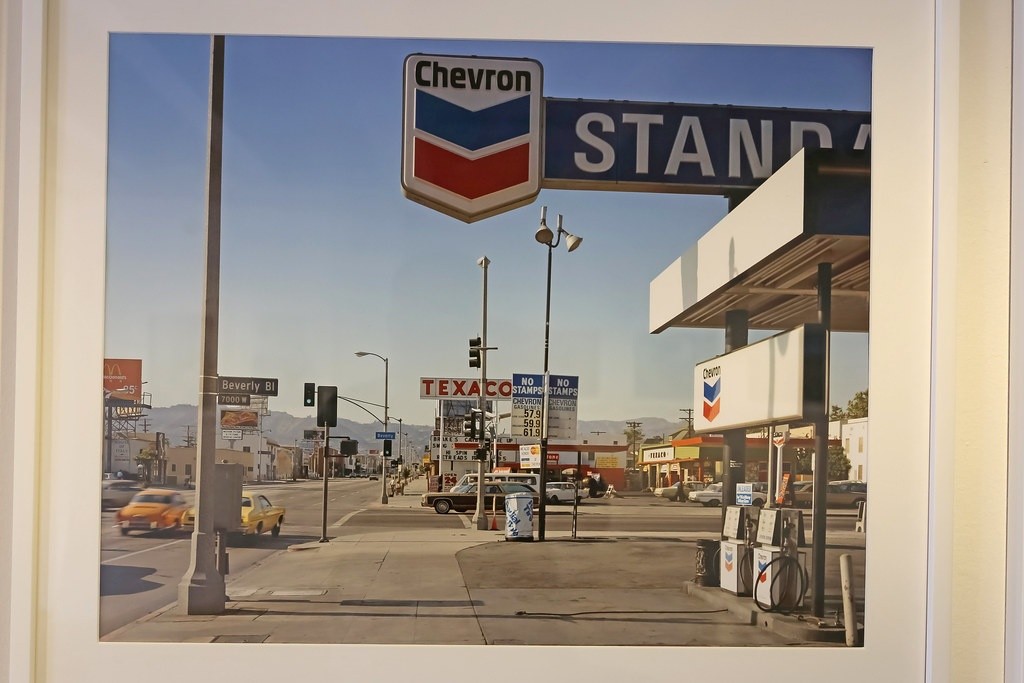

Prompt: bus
xmin=450 ymin=473 xmax=540 ymax=494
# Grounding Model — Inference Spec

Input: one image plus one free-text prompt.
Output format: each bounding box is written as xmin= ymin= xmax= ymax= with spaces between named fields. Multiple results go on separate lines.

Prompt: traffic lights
xmin=485 ymin=438 xmax=491 ymax=451
xmin=464 ymin=411 xmax=476 ymax=438
xmin=469 ymin=337 xmax=481 ymax=368
xmin=384 ymin=440 xmax=392 ymax=456
xmin=304 ymin=383 xmax=315 ymax=406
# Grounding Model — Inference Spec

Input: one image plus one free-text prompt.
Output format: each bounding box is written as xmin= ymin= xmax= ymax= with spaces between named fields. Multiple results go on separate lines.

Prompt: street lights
xmin=396 ymin=431 xmax=423 ymax=484
xmin=354 ymin=351 xmax=389 ymax=504
xmin=387 ymin=416 xmax=403 ymax=484
xmin=535 ymin=206 xmax=583 ymax=543
xmin=471 ymin=255 xmax=491 ymax=530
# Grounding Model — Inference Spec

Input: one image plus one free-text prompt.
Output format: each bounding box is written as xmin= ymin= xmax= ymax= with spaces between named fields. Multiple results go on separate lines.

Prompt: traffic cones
xmin=490 ymin=514 xmax=499 ymax=530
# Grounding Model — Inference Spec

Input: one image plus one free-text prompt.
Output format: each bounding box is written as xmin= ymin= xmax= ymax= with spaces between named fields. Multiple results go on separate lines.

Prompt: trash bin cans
xmin=696 ymin=539 xmax=720 ymax=587
xmin=505 ymin=492 xmax=534 ymax=542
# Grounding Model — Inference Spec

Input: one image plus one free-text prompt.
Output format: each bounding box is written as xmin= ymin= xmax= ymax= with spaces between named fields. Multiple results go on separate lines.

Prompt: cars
xmin=546 ymin=481 xmax=589 ymax=505
xmin=350 ymin=471 xmax=378 ymax=481
xmin=180 ymin=491 xmax=286 ymax=541
xmin=421 ymin=481 xmax=540 ymax=514
xmin=115 ymin=489 xmax=188 ymax=535
xmin=653 ymin=480 xmax=867 ymax=509
xmin=102 ymin=479 xmax=145 ymax=511
